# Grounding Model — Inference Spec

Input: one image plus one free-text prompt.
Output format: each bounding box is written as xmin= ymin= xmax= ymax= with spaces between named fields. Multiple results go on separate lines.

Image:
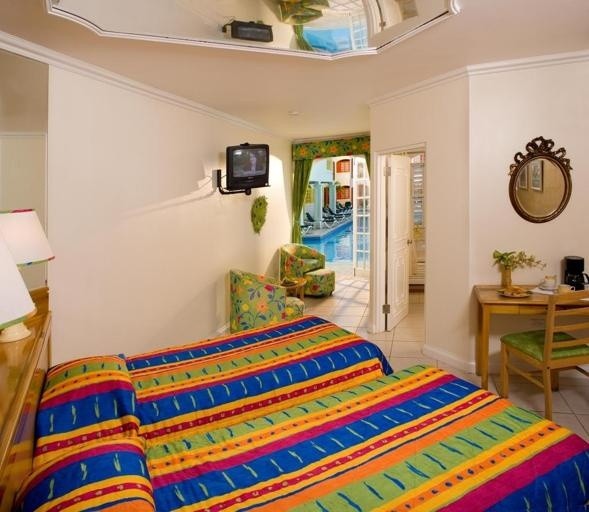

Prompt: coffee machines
xmin=564 ymin=256 xmax=589 ymax=291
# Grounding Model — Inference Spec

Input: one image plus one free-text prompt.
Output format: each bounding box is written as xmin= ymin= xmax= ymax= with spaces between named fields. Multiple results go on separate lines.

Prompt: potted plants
xmin=494 ymin=250 xmax=548 ymax=287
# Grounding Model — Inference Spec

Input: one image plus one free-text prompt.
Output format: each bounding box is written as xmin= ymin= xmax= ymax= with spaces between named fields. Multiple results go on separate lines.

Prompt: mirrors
xmin=510 ymin=135 xmax=575 ymax=223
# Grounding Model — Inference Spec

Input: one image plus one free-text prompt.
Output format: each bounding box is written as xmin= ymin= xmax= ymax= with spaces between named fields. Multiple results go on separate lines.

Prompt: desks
xmin=473 ymin=285 xmax=589 ymax=392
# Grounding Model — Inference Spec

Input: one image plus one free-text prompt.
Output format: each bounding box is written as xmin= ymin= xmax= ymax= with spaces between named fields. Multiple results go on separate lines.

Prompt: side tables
xmin=276 ymin=276 xmax=305 ymax=297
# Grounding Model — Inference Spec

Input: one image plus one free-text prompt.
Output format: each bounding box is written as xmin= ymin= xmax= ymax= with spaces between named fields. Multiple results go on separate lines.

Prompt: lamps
xmin=0 ymin=254 xmax=37 ymax=328
xmin=0 ymin=209 xmax=55 ymax=342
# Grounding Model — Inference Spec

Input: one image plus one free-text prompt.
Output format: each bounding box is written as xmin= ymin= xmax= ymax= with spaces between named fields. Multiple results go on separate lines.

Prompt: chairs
xmin=279 ymin=242 xmax=333 ymax=297
xmin=501 ymin=290 xmax=589 ymax=421
xmin=280 ymin=1 xmax=317 ymax=23
xmin=230 ymin=268 xmax=303 ymax=334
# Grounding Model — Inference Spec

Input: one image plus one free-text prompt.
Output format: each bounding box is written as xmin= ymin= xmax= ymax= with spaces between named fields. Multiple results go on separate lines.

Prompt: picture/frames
xmin=517 ymin=166 xmax=527 ymax=189
xmin=530 ymin=159 xmax=543 ymax=191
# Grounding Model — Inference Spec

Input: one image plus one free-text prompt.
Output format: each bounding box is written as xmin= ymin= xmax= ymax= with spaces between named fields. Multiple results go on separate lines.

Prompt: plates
xmin=539 ymin=284 xmax=559 ymax=290
xmin=496 ymin=293 xmax=531 ymax=298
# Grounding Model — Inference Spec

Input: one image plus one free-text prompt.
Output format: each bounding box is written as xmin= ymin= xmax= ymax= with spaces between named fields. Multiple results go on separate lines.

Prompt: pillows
xmin=14 ymin=441 xmax=155 ymax=511
xmin=34 ymin=354 xmax=141 ymax=464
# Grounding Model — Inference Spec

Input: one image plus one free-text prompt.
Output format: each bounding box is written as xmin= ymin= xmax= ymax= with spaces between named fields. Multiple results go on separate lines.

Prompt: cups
xmin=558 ymin=285 xmax=576 ymax=293
xmin=541 ymin=278 xmax=555 ymax=289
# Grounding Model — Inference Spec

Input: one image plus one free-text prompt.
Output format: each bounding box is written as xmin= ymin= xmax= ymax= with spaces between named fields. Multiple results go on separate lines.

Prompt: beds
xmin=11 ymin=363 xmax=589 ymax=511
xmin=27 ymin=315 xmax=396 ymax=473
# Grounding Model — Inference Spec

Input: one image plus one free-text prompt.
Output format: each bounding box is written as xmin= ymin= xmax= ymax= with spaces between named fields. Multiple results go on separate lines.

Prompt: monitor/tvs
xmin=222 ymin=20 xmax=274 ymax=44
xmin=217 ymin=143 xmax=270 ymax=195
